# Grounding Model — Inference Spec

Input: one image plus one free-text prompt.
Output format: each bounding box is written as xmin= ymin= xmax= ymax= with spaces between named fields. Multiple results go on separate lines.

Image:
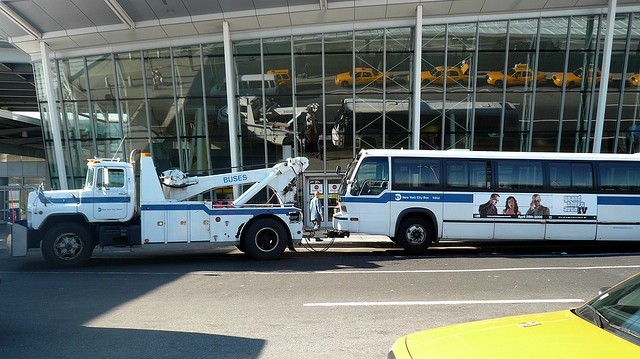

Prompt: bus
xmin=217 ymin=96 xmax=320 ymax=156
xmin=332 ymin=97 xmax=518 ymax=150
xmin=332 ymin=147 xmax=640 ymax=253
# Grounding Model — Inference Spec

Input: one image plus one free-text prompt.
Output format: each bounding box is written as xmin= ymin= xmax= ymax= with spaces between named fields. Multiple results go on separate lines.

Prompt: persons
xmin=526 ymin=194 xmax=550 ymax=215
xmin=316 ymin=134 xmax=324 ymax=162
xmin=307 ymin=191 xmax=324 ymax=242
xmin=503 ymin=196 xmax=521 ymax=216
xmin=479 ymin=194 xmax=500 ymax=217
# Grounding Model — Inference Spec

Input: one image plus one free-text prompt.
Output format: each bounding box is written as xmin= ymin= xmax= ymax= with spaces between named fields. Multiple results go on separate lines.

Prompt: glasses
xmin=532 ymin=199 xmax=541 ymax=202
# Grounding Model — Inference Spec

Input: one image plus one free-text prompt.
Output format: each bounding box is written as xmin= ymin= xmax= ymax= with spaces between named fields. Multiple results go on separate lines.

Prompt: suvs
xmin=210 ymin=74 xmax=278 ymax=101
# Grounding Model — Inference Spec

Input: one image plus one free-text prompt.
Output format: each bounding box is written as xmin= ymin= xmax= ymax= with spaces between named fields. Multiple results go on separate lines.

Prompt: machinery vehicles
xmin=10 ymin=146 xmax=309 ymax=269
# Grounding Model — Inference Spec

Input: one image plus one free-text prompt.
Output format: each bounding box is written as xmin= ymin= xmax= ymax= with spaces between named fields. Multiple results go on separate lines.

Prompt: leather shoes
xmin=306 ymin=236 xmax=310 ymax=241
xmin=316 ymin=239 xmax=323 ymax=241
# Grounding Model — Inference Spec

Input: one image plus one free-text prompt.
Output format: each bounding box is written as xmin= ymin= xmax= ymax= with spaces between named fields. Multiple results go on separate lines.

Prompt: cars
xmin=385 ymin=270 xmax=640 ymax=359
xmin=265 ymin=70 xmax=292 ymax=95
xmin=486 ymin=63 xmax=547 ymax=88
xmin=552 ymin=64 xmax=613 ymax=90
xmin=406 ymin=63 xmax=472 ymax=86
xmin=335 ymin=68 xmax=392 ymax=88
xmin=628 ymin=68 xmax=640 ymax=86
